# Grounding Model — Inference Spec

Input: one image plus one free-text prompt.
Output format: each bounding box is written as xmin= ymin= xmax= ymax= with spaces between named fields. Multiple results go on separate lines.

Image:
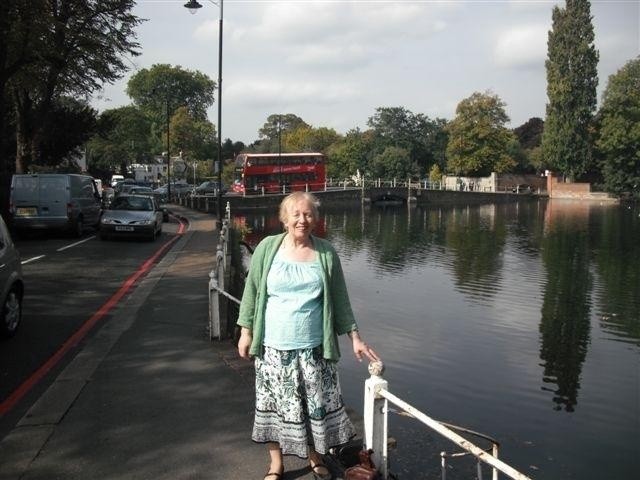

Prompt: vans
xmin=8 ymin=171 xmax=106 ymax=242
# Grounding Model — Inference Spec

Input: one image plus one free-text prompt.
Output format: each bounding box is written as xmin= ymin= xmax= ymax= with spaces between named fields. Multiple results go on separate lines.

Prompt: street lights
xmin=268 ymin=116 xmax=289 ymax=194
xmin=183 ymin=0 xmax=224 ymax=234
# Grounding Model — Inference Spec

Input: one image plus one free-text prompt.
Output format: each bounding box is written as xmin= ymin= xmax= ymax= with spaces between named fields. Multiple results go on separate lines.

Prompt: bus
xmin=231 ymin=152 xmax=326 ymax=195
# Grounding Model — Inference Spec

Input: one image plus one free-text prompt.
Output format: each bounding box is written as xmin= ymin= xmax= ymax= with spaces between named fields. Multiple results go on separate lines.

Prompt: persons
xmin=236 ymin=192 xmax=381 ymax=480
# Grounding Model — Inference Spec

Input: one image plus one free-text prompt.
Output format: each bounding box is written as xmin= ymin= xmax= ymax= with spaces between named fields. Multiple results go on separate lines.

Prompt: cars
xmin=192 ymin=180 xmax=228 ymax=197
xmin=1 ymin=214 xmax=23 ymax=339
xmin=511 ymin=183 xmax=536 ymax=195
xmin=153 ymin=182 xmax=193 ymax=203
xmin=90 ymin=173 xmax=169 ymax=221
xmin=99 ymin=192 xmax=164 ymax=243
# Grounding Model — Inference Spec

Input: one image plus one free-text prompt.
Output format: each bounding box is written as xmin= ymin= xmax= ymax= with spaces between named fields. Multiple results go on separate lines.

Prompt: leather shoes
xmin=262 ymin=464 xmax=284 ymax=480
xmin=309 ymin=457 xmax=333 ymax=480
